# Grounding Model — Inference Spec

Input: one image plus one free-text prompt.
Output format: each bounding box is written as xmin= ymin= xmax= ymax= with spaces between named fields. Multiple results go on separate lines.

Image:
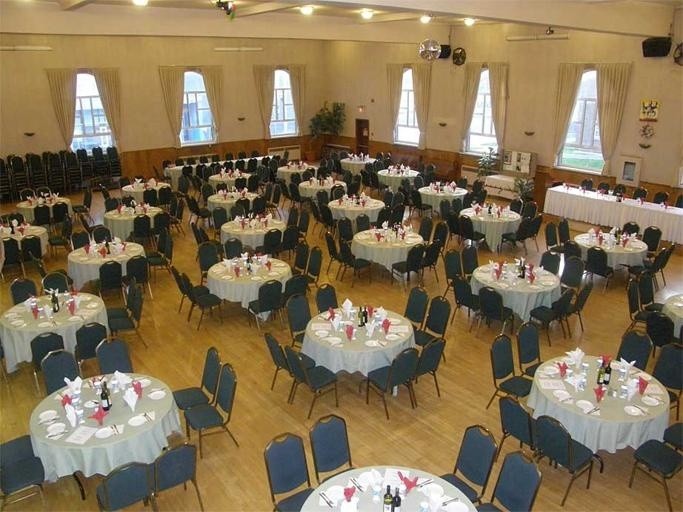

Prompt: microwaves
xmin=356 ymin=193 xmax=360 ymax=204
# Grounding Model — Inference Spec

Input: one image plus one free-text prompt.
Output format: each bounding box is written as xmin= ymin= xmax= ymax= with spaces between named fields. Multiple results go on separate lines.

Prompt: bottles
xmin=8 ymin=221 xmax=15 ymax=234
xmin=488 ymin=203 xmax=493 ymax=214
xmin=104 ymin=240 xmax=111 ymax=254
xmin=396 ymin=160 xmax=400 ymax=173
xmin=440 ymin=177 xmax=444 ymax=191
xmin=131 ymin=202 xmax=135 ymax=213
xmin=616 ymin=227 xmax=620 ymax=245
xmin=598 ymin=230 xmax=604 ymax=244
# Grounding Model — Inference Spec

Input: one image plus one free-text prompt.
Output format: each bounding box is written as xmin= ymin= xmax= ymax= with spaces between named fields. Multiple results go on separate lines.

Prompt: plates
xmin=314 ymin=307 xmax=401 ymax=347
xmin=545 ymin=357 xmax=661 ymax=416
xmin=357 ymin=229 xmax=418 ymax=248
xmin=481 ymin=262 xmax=555 ymax=290
xmin=38 ymin=377 xmax=166 ymax=437
xmin=4 ymin=295 xmax=99 ymax=328
xmin=223 ymin=218 xmax=283 ymax=233
xmin=319 ymin=471 xmax=469 ymax=512
xmin=211 ymin=257 xmax=286 ymax=281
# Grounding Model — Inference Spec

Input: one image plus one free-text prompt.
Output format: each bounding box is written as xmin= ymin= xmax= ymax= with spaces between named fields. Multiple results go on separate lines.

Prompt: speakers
xmin=439 ymin=45 xmax=452 ymax=58
xmin=642 ymin=37 xmax=672 ymax=57
